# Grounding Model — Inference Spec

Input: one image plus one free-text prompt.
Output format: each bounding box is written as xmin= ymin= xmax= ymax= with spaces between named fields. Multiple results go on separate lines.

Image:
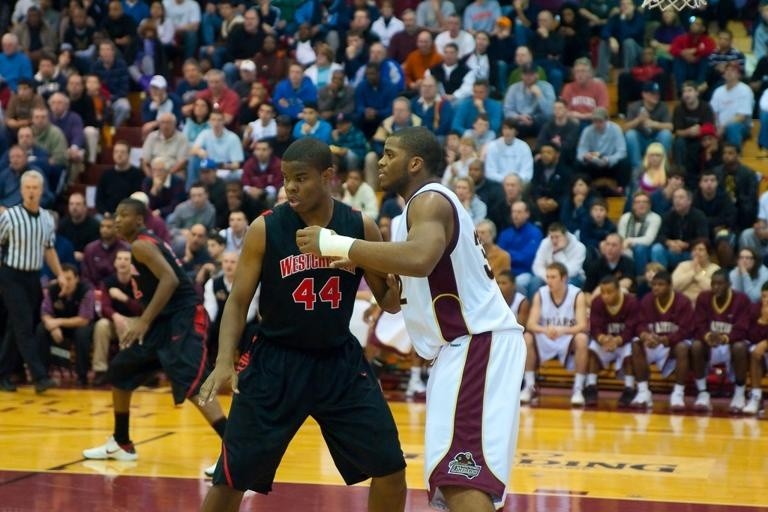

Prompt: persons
xmin=197 ymin=138 xmax=408 ymax=512
xmin=84 ymin=198 xmax=228 ymax=478
xmin=294 ymin=124 xmax=529 ymax=512
xmin=0 ymin=1 xmax=768 ymax=414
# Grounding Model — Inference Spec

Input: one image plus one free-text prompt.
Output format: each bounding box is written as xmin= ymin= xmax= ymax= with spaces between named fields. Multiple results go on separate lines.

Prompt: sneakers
xmin=407 ymin=378 xmax=761 ymax=416
xmin=82 ymin=438 xmax=136 ymax=461
xmin=35 ymin=377 xmax=54 ymax=392
xmin=0 ymin=375 xmax=17 ymax=392
xmin=206 ymin=462 xmax=216 ymax=475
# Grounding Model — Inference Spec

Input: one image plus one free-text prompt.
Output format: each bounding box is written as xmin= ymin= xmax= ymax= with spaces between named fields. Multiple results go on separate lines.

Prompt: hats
xmin=497 ymin=17 xmax=511 ymax=28
xmin=151 ymin=75 xmax=167 ymax=88
xmin=240 ymin=60 xmax=256 ymax=71
xmin=336 ymin=113 xmax=350 ymax=123
xmin=523 ymin=63 xmax=537 ymax=73
xmin=642 ymin=80 xmax=661 ymax=92
xmin=701 ymin=124 xmax=717 ymax=137
xmin=200 ymin=158 xmax=217 ymax=169
xmin=592 ymin=108 xmax=609 ymax=120
